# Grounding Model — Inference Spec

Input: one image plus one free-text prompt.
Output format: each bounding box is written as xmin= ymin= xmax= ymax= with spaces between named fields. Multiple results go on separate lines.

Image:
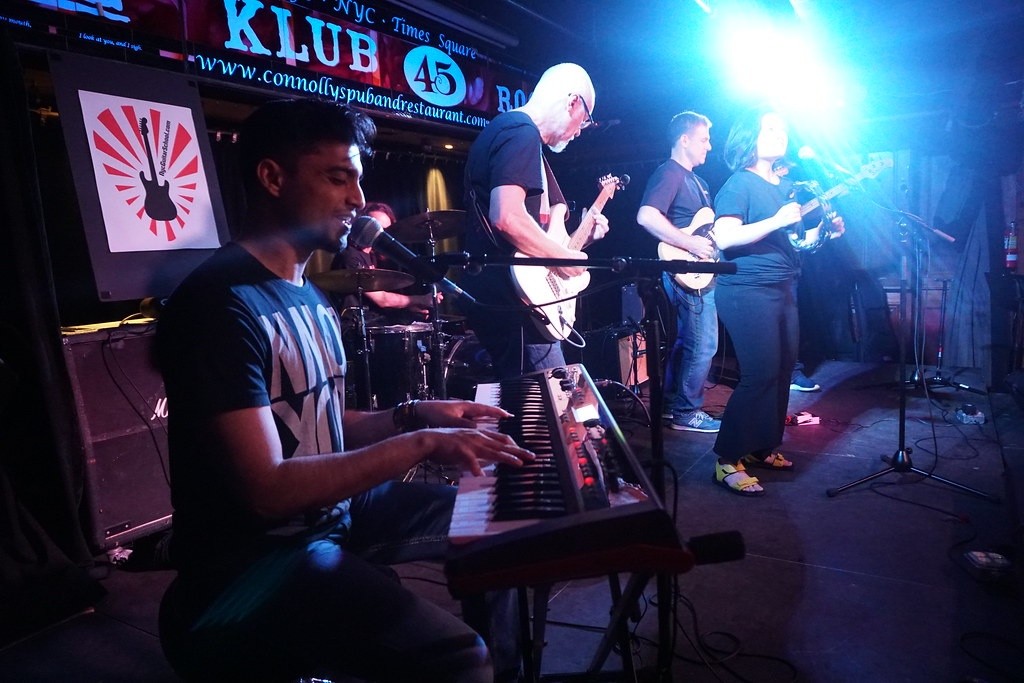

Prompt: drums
xmin=347 ymin=321 xmax=435 ymax=411
xmin=429 ymin=332 xmax=494 ymax=402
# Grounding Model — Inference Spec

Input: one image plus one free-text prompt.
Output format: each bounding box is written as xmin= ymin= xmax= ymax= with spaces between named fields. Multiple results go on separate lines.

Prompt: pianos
xmin=440 ymin=359 xmax=698 ymax=604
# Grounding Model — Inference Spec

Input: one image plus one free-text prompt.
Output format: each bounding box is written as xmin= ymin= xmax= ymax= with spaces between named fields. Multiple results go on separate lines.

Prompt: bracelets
xmin=393 ymin=400 xmax=428 ymax=433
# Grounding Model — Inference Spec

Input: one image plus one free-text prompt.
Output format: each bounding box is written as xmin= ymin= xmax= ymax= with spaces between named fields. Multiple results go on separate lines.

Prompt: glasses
xmin=568 ymin=93 xmax=595 ymax=129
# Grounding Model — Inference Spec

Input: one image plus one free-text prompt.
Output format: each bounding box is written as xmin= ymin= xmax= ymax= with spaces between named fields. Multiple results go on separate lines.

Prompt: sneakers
xmin=661 ymin=408 xmax=673 ymax=419
xmin=790 ymin=372 xmax=820 ymax=391
xmin=671 ymin=409 xmax=722 ymax=433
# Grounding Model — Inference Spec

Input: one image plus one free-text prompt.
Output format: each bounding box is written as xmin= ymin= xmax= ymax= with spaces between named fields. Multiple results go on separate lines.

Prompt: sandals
xmin=713 ymin=457 xmax=766 ymax=497
xmin=743 ymin=451 xmax=796 ymax=471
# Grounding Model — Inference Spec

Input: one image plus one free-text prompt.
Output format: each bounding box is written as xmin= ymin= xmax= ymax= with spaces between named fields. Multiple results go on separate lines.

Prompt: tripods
xmin=824 ymin=174 xmax=988 ymax=500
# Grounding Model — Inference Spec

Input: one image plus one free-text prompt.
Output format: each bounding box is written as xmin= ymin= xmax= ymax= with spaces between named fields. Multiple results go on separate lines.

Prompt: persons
xmin=330 ymin=201 xmax=444 ymax=411
xmin=634 ymin=104 xmax=845 ymax=493
xmin=151 ymin=94 xmax=535 ymax=683
xmin=463 ymin=62 xmax=608 ymax=372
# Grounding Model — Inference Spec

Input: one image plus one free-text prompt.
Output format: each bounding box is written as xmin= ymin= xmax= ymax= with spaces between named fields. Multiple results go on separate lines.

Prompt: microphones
xmin=630 ymin=350 xmax=646 ymax=357
xmin=349 ymin=214 xmax=475 ymax=303
xmin=797 ymin=145 xmax=851 ymax=175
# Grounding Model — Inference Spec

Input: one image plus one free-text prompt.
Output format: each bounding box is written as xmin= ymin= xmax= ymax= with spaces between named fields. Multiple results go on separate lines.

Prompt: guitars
xmin=504 ymin=171 xmax=626 ymax=345
xmin=799 ymin=157 xmax=894 ymax=218
xmin=655 ymin=205 xmax=717 ymax=293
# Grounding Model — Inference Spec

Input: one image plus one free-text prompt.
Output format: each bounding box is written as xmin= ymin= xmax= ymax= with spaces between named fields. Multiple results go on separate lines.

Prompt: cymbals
xmin=387 ymin=208 xmax=470 ymax=247
xmin=308 ymin=262 xmax=416 ymax=297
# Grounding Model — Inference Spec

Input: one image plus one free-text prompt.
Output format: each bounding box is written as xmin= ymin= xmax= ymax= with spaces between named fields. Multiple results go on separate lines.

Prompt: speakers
xmin=579 ymin=324 xmax=651 ymax=391
xmin=58 ymin=327 xmax=176 ymax=551
